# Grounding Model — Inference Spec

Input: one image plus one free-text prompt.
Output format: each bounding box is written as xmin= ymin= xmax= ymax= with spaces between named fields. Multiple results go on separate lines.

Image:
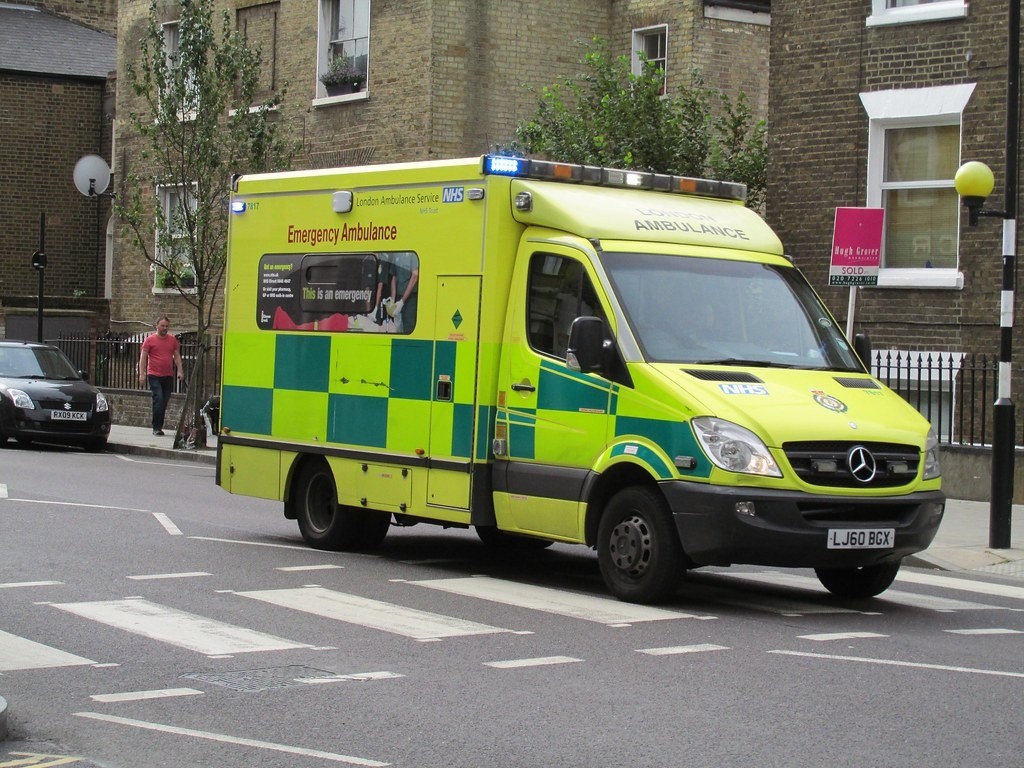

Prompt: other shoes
xmin=153 ymin=428 xmax=165 ymax=436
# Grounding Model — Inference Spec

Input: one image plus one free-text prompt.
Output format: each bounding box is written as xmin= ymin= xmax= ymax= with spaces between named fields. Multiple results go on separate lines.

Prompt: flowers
xmin=318 ymin=49 xmax=367 ymax=87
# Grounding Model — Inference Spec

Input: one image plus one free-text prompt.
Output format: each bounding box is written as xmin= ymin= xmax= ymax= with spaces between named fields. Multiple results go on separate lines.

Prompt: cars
xmin=0 ymin=339 xmax=110 ymax=452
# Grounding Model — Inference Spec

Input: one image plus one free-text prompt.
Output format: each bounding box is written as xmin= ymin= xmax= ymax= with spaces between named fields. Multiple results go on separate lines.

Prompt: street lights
xmin=955 ymin=161 xmax=1015 ymax=549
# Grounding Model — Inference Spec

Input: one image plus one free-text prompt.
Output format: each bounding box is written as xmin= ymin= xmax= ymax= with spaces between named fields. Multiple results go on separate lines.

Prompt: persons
xmin=389 ymin=251 xmax=419 ymax=334
xmin=362 ymin=252 xmax=391 ymax=325
xmin=139 ymin=316 xmax=186 ymax=436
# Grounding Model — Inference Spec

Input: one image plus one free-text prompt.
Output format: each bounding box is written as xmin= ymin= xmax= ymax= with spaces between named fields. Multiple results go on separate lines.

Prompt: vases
xmin=326 ymin=82 xmax=360 ymax=97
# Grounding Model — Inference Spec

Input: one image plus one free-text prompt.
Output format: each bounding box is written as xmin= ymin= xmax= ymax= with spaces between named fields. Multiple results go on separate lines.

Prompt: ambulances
xmin=215 ymin=153 xmax=945 ymax=603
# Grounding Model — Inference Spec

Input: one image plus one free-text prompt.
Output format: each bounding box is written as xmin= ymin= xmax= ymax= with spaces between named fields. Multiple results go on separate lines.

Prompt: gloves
xmin=370 ymin=306 xmax=378 ymax=321
xmin=393 ymin=299 xmax=404 ymax=316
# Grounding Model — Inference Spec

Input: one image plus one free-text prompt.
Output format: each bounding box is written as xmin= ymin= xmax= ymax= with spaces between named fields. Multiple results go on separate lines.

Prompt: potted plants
xmin=156 ymin=267 xmax=194 ymax=290
